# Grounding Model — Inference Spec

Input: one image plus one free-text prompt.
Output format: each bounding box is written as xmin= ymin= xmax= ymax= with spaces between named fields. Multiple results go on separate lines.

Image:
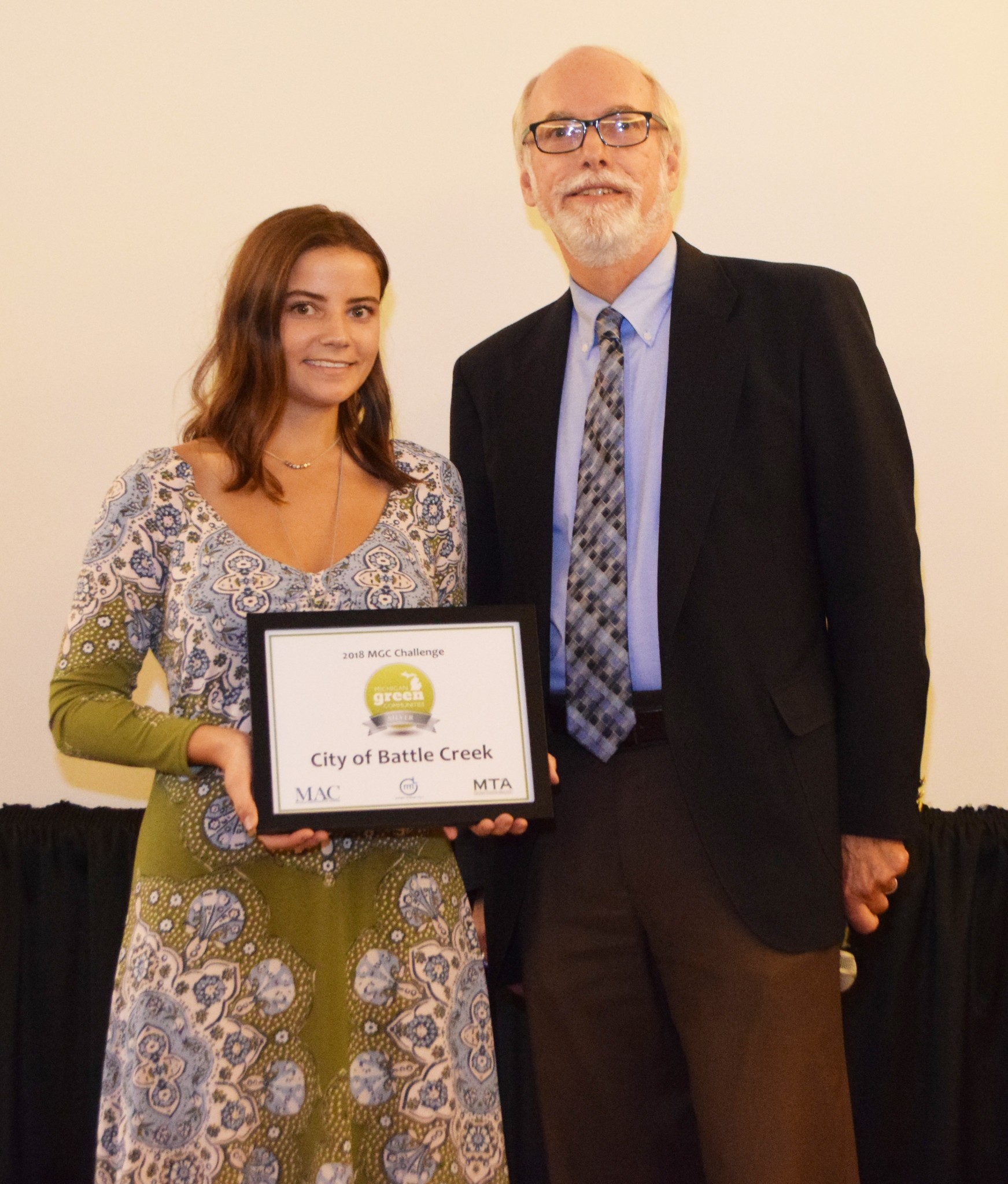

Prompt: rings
xmin=885 ymin=878 xmax=897 ymax=894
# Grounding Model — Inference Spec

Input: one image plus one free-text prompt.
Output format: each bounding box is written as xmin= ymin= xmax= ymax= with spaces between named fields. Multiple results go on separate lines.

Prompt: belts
xmin=547 ymin=709 xmax=668 ymax=750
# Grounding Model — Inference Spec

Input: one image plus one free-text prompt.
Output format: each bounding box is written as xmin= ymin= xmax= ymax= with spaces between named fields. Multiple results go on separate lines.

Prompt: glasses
xmin=520 ymin=111 xmax=669 ymax=154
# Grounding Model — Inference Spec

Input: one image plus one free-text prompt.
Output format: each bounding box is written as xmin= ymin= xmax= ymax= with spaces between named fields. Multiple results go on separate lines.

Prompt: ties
xmin=564 ymin=304 xmax=636 ymax=763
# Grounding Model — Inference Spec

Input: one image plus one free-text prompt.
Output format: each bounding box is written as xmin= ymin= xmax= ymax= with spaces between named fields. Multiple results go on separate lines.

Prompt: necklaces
xmin=263 ymin=435 xmax=342 ymax=468
xmin=274 ymin=442 xmax=344 ymax=611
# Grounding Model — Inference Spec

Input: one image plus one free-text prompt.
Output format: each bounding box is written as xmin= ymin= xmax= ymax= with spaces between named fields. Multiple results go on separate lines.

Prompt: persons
xmin=48 ymin=204 xmax=559 ymax=1184
xmin=449 ymin=48 xmax=930 ymax=1183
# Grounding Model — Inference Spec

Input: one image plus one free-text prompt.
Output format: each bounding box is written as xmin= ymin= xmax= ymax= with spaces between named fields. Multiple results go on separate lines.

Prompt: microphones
xmin=838 ymin=923 xmax=857 ymax=991
